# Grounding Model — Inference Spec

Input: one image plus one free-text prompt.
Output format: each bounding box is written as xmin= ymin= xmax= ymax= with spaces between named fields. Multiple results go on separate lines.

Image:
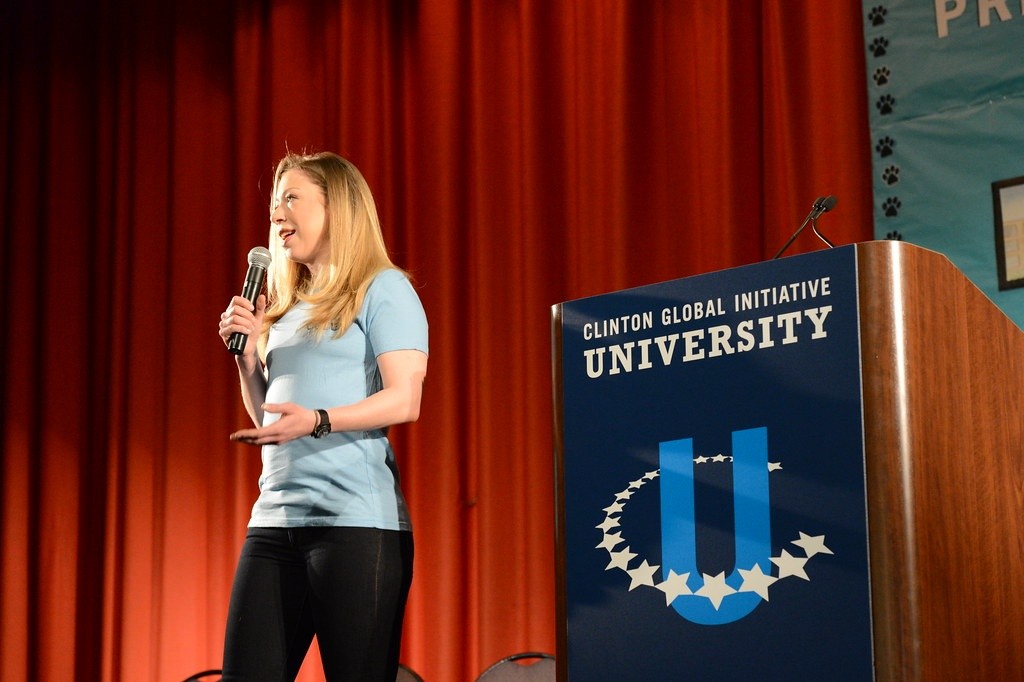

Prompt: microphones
xmin=773 ymin=195 xmax=837 ymax=260
xmin=227 ymin=246 xmax=272 ymax=355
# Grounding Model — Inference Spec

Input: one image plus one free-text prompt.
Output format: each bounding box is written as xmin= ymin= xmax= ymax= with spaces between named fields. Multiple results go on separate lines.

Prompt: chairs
xmin=474 ymin=650 xmax=556 ymax=681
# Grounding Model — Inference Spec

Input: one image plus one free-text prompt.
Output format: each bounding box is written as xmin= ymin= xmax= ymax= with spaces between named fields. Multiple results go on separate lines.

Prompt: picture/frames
xmin=991 ymin=176 xmax=1024 ymax=292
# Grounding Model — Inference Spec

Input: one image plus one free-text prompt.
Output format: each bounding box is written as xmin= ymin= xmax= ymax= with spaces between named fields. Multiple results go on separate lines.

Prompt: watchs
xmin=310 ymin=409 xmax=331 ymax=439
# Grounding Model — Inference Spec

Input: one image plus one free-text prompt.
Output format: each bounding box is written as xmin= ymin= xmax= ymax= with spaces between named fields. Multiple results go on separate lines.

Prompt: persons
xmin=219 ymin=151 xmax=429 ymax=682
